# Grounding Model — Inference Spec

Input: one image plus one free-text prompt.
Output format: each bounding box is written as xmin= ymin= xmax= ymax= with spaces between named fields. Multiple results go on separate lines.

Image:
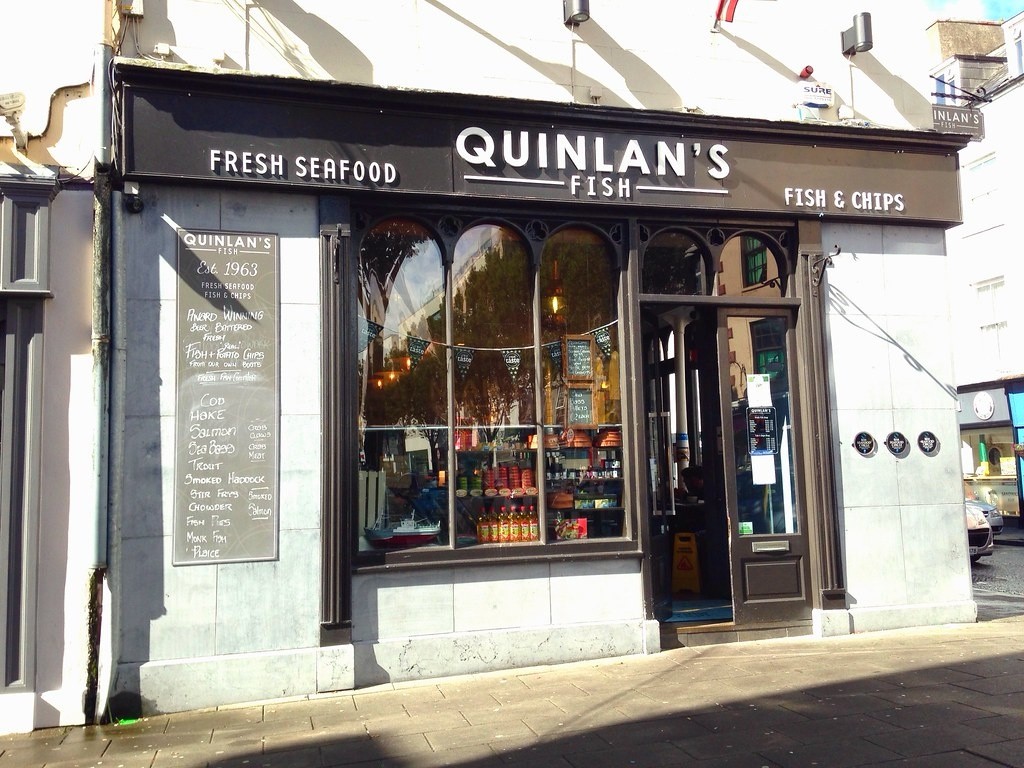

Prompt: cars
xmin=964 ymin=503 xmax=992 ymax=565
xmin=974 ymin=498 xmax=1004 ymax=536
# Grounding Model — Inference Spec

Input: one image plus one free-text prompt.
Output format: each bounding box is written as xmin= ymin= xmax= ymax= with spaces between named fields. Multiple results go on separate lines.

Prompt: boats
xmin=362 ymin=509 xmax=441 ymax=547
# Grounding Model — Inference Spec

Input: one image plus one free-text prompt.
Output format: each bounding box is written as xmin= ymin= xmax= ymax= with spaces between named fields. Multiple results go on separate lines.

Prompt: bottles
xmin=476 ymin=507 xmax=489 ymax=543
xmin=488 ymin=507 xmax=499 ymax=543
xmin=509 ymin=507 xmax=521 ymax=541
xmin=519 ymin=506 xmax=530 ymax=541
xmin=499 ymin=506 xmax=509 ymax=542
xmin=528 ymin=505 xmax=539 ymax=541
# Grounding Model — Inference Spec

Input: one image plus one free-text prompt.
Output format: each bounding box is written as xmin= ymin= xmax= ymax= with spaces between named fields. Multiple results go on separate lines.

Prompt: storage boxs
xmin=546 ymin=450 xmax=618 ymax=540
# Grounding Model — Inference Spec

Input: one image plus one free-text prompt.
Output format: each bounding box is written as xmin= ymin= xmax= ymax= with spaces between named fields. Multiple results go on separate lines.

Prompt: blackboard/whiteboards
xmin=172 ymin=228 xmax=279 ymax=566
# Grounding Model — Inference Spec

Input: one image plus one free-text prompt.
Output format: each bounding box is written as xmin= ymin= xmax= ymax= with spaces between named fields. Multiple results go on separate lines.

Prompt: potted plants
xmin=1014 ymin=444 xmax=1024 ymax=457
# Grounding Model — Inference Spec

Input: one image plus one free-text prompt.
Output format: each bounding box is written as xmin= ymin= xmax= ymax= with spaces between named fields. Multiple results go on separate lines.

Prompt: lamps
xmin=840 ymin=12 xmax=873 ymax=55
xmin=563 ymin=0 xmax=589 ymax=26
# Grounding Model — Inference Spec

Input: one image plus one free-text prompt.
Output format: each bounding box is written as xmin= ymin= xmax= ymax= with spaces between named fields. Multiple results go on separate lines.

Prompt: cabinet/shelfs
xmin=544 ymin=445 xmax=626 ymax=512
xmin=455 ymin=449 xmax=539 ymax=499
